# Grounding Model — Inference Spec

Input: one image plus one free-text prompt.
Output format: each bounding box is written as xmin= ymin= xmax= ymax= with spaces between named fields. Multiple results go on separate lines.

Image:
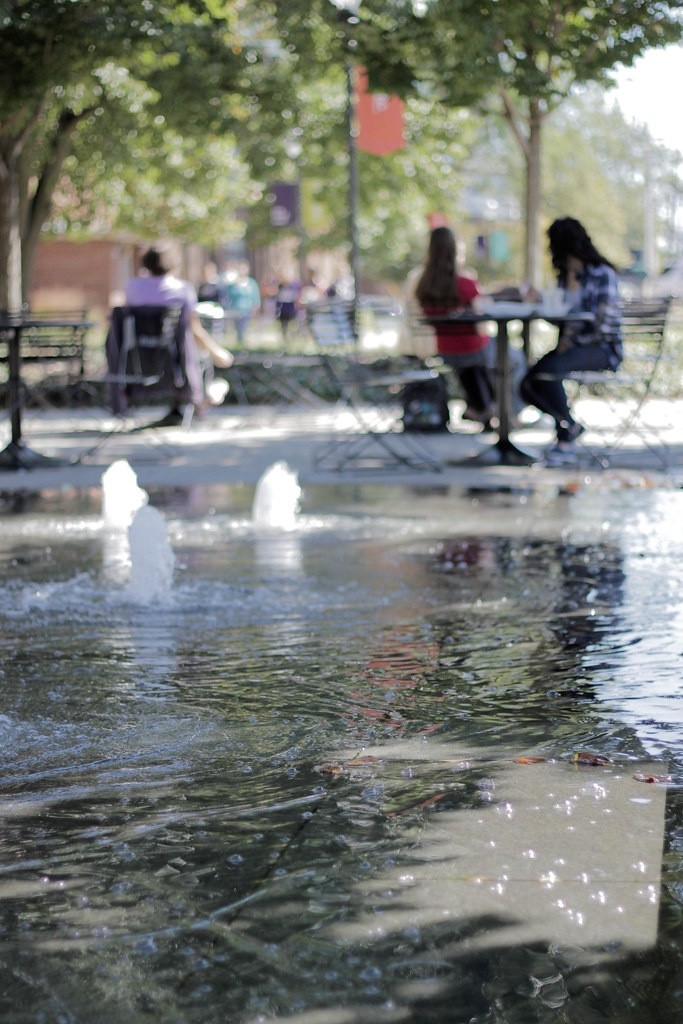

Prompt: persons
xmin=274 ymin=263 xmax=355 ymax=341
xmin=515 ymin=217 xmax=622 ymax=452
xmin=227 ymin=257 xmax=260 ymax=341
xmin=198 ymin=262 xmax=229 ymax=341
xmin=409 ymin=228 xmax=543 ymax=425
xmin=118 ymin=243 xmax=234 ymax=406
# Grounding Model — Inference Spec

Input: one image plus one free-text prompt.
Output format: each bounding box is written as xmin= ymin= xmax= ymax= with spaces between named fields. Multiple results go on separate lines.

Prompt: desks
xmin=0 ymin=321 xmax=96 ymax=470
xmin=417 ymin=313 xmax=595 ymax=466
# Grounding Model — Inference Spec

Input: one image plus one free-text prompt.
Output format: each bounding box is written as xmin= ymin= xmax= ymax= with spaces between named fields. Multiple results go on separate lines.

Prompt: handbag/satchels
xmin=401 ymin=373 xmax=450 ymax=433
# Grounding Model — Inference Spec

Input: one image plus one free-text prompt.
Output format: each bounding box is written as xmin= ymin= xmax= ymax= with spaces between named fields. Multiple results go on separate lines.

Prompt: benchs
xmin=3 ymin=309 xmax=89 ymax=375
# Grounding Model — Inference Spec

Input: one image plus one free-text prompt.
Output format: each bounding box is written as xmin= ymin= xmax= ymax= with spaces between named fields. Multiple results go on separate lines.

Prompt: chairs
xmin=65 ymin=302 xmax=183 ymax=465
xmin=538 ymin=294 xmax=676 ymax=472
xmin=305 ymin=300 xmax=440 ymax=473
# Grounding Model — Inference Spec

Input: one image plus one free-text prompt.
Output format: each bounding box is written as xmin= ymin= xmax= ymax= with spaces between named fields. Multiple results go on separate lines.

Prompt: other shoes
xmin=545 ymin=439 xmax=578 ymax=464
xmin=208 ymin=376 xmax=231 ymax=404
xmin=463 ymin=409 xmax=493 ymax=422
